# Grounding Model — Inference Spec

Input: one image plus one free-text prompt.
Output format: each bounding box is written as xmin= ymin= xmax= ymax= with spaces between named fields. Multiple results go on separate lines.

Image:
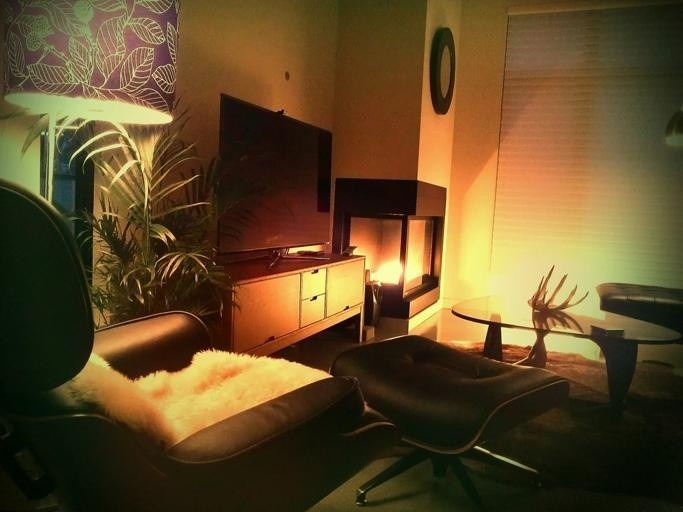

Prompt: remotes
xmin=297 ymin=250 xmax=325 ymax=257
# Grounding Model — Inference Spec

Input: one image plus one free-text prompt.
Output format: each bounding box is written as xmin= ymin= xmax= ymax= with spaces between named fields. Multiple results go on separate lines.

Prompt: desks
xmin=200 ymin=252 xmax=368 ymax=363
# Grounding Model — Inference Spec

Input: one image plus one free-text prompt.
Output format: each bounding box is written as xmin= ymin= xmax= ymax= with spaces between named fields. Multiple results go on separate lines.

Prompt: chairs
xmin=0 ymin=178 xmax=403 ymax=512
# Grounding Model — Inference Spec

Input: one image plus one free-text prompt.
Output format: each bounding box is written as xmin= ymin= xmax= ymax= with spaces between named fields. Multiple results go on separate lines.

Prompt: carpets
xmin=441 ymin=344 xmax=681 ymax=497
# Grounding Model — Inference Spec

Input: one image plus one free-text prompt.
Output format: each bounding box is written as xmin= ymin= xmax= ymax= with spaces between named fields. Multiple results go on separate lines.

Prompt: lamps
xmin=0 ymin=0 xmax=183 ymax=208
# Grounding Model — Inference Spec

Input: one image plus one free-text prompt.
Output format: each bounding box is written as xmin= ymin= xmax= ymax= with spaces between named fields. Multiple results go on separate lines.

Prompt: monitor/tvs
xmin=216 ymin=92 xmax=333 ymax=271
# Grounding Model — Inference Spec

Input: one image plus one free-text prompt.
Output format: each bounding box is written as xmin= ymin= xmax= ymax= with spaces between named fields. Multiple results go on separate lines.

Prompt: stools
xmin=329 ymin=334 xmax=570 ymax=512
xmin=594 ymin=279 xmax=682 ymax=370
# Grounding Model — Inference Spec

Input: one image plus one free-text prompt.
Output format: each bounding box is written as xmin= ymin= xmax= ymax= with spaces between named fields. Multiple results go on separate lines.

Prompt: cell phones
xmin=591 ymin=323 xmax=625 ymax=334
